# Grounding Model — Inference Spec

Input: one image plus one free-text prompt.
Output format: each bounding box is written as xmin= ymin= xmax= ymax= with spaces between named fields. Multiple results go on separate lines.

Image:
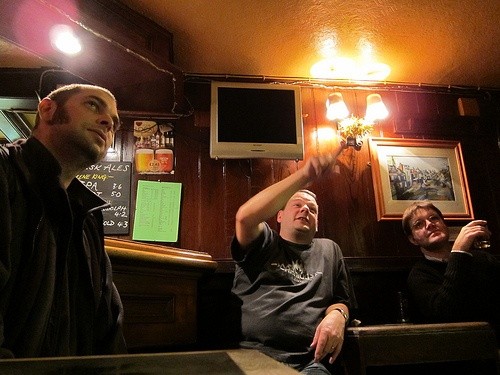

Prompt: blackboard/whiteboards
xmin=74 ymin=158 xmax=132 ymax=234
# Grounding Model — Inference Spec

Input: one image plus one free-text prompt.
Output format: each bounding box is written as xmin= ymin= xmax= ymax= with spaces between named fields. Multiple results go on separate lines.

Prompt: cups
xmin=474 ymin=223 xmax=492 ymax=249
xmin=156 ymin=149 xmax=174 ymax=172
xmin=135 ymin=149 xmax=154 ymax=172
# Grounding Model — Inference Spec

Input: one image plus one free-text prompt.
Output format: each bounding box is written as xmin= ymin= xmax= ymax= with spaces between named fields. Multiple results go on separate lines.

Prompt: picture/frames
xmin=369 ymin=137 xmax=475 ymax=223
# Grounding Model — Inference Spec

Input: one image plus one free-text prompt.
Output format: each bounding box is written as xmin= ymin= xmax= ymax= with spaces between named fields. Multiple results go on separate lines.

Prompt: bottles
xmin=136 ymin=132 xmax=173 ymax=149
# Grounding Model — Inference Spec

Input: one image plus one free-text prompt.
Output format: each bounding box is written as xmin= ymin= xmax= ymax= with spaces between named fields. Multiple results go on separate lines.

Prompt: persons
xmin=415 ymin=164 xmax=451 ymax=189
xmin=0 ymin=84 xmax=127 ymax=359
xmin=402 ymin=201 xmax=495 ymax=324
xmin=221 ymin=143 xmax=356 ymax=375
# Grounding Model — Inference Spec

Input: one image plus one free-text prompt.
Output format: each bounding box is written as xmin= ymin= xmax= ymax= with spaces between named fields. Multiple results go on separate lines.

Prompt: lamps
xmin=326 ymin=92 xmax=388 ymax=149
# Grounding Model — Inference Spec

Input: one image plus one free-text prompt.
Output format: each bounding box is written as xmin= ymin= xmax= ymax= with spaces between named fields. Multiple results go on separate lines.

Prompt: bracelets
xmin=333 ymin=307 xmax=348 ymax=320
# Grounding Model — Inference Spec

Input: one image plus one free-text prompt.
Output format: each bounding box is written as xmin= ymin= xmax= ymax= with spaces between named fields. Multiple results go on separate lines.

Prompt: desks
xmin=344 ymin=322 xmax=499 ymax=375
xmin=0 ymin=347 xmax=301 ymax=375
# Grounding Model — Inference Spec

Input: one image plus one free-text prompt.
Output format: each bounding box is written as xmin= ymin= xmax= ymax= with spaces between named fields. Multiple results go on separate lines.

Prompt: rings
xmin=331 ymin=346 xmax=335 ymax=349
